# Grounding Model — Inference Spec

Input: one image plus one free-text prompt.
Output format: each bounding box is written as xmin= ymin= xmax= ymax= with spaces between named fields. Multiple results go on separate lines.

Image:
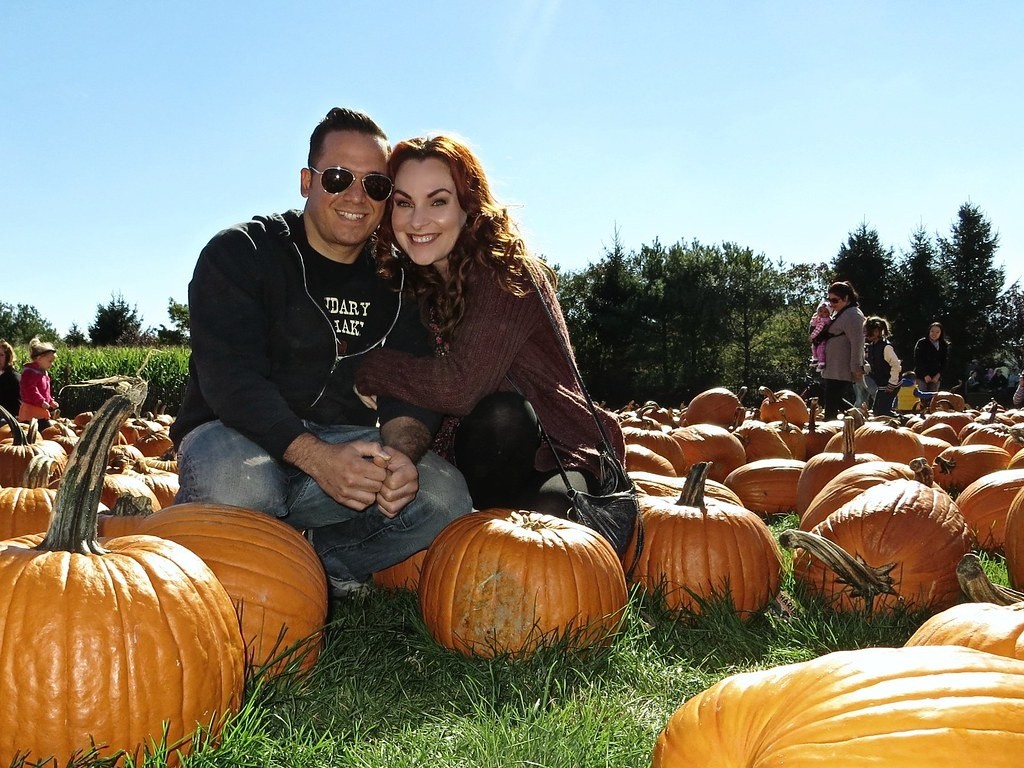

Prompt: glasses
xmin=309 ymin=166 xmax=394 ymax=203
xmin=826 ymin=297 xmax=843 ymax=303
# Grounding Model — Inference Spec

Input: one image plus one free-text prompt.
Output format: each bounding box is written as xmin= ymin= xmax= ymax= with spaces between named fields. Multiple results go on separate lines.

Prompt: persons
xmin=991 ymin=369 xmax=1008 ymax=410
xmin=1010 ymin=368 xmax=1024 ymax=405
xmin=865 ymin=317 xmax=903 ymax=418
xmin=170 ymin=107 xmax=473 ymax=596
xmin=19 ymin=343 xmax=60 ymax=430
xmin=811 ymin=279 xmax=865 ymax=420
xmin=355 ymin=139 xmax=625 ymax=521
xmin=968 ymin=371 xmax=978 ymax=392
xmin=914 ymin=323 xmax=949 ymax=409
xmin=0 ymin=339 xmax=19 ymax=422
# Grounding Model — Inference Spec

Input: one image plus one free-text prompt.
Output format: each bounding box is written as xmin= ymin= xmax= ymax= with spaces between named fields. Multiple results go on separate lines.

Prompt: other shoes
xmin=816 ymin=361 xmax=825 ymax=372
xmin=809 ymin=359 xmax=818 ymax=366
xmin=329 ymin=581 xmax=371 ymax=600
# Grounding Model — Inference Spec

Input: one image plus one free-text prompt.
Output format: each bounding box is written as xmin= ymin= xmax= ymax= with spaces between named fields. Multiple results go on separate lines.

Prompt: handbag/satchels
xmin=571 ymin=454 xmax=637 ymax=558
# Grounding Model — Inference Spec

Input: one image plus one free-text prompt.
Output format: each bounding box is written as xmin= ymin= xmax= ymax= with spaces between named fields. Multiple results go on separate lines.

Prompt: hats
xmin=816 ymin=297 xmax=834 ymax=317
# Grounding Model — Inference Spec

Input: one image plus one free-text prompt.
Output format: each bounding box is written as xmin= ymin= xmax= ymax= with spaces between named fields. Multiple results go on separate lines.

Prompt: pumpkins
xmin=0 ymin=392 xmax=329 ymax=768
xmin=365 ymin=386 xmax=1024 ymax=768
